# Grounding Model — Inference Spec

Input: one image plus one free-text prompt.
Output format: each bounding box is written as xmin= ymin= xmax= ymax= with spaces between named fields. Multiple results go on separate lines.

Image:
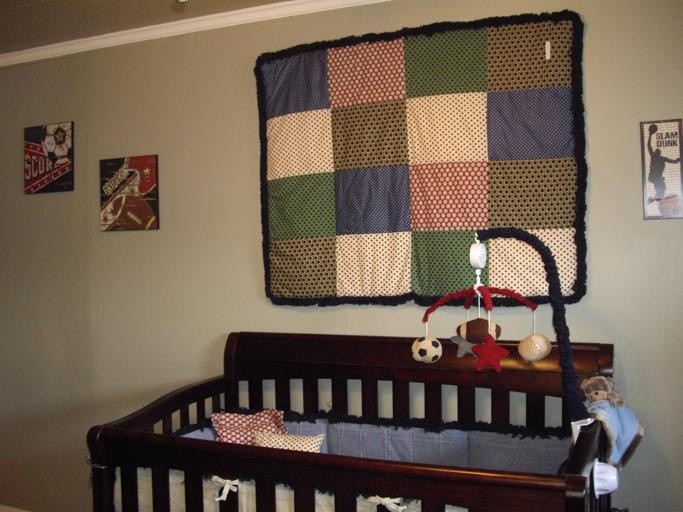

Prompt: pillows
xmin=210 ymin=409 xmax=326 ymax=454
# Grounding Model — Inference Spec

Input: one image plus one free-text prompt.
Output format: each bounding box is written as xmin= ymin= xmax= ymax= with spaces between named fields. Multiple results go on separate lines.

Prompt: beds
xmin=84 ymin=334 xmax=615 ymax=510
